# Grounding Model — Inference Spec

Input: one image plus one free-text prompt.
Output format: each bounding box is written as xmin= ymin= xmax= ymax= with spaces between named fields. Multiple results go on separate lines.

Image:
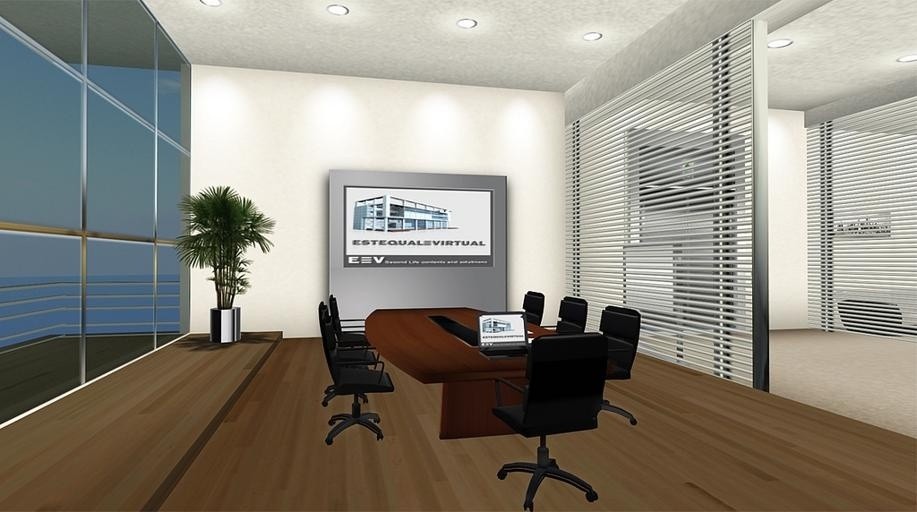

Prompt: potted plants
xmin=177 ymin=187 xmax=275 ymax=344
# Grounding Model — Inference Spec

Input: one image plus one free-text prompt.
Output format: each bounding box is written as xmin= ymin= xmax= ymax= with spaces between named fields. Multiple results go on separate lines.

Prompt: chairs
xmin=836 ymin=298 xmax=902 ymax=334
xmin=489 ymin=331 xmax=608 ymax=511
xmin=523 ymin=289 xmax=641 ymax=427
xmin=318 ymin=295 xmax=393 ymax=445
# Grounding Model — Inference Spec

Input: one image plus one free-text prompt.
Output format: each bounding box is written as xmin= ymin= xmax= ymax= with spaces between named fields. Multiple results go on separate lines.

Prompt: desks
xmin=365 ymin=306 xmax=556 ymax=439
